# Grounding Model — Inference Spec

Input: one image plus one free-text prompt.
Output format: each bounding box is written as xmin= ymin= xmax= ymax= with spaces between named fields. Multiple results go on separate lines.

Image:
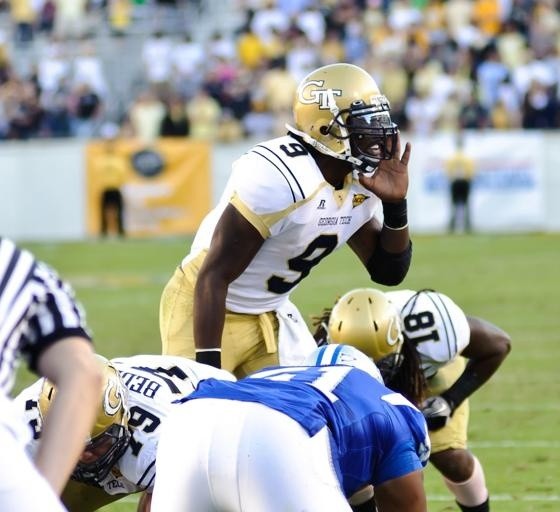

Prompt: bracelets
xmin=194 ymin=347 xmax=223 ymax=369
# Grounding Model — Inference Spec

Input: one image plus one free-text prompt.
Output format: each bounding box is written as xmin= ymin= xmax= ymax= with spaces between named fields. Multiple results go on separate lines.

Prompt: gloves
xmin=418 ymin=394 xmax=452 ymax=433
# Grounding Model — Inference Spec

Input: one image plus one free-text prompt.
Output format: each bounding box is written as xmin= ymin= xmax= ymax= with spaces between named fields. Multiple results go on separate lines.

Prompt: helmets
xmin=291 ymin=62 xmax=398 ymax=175
xmin=303 ymin=343 xmax=385 ymax=386
xmin=326 ymin=288 xmax=404 ymax=375
xmin=38 ymin=351 xmax=131 ymax=484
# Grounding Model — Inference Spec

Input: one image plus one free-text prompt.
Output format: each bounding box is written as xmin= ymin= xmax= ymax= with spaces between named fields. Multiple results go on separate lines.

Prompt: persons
xmin=446 ymin=140 xmax=476 ymax=235
xmin=11 ymin=352 xmax=236 ymax=511
xmin=94 ymin=139 xmax=127 ymax=236
xmin=0 ymin=237 xmax=110 ymax=512
xmin=1 ymin=0 xmax=559 ymax=140
xmin=311 ymin=286 xmax=512 ymax=512
xmin=149 ymin=340 xmax=434 ymax=512
xmin=156 ymin=60 xmax=416 ymax=384
